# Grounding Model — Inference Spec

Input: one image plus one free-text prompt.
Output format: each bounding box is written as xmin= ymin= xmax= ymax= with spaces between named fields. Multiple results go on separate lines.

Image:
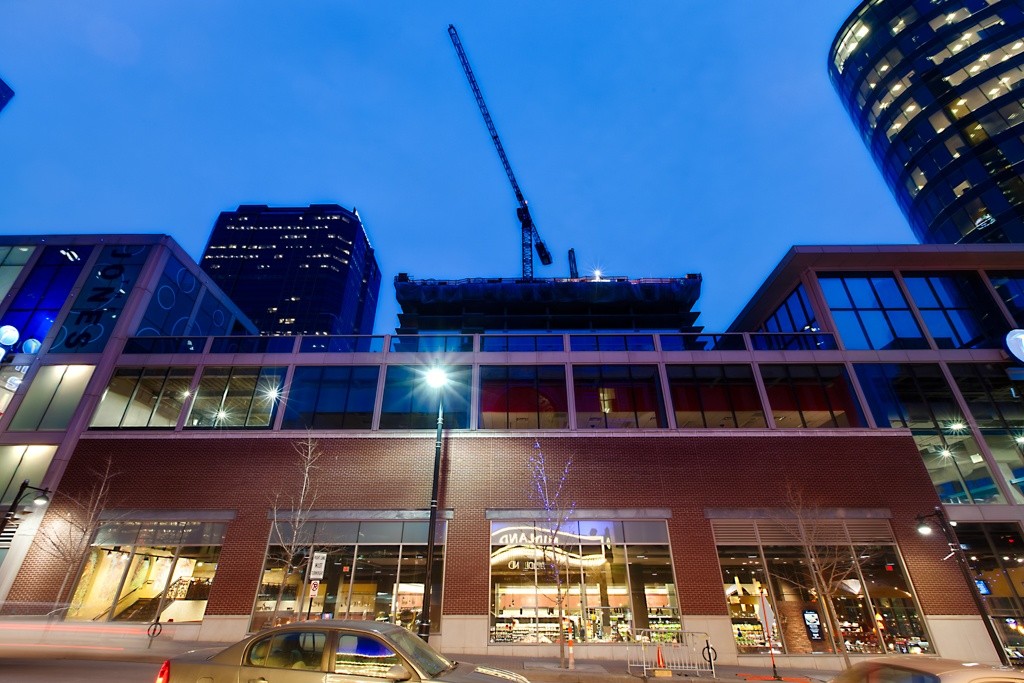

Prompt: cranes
xmin=446 ymin=21 xmax=554 ymax=281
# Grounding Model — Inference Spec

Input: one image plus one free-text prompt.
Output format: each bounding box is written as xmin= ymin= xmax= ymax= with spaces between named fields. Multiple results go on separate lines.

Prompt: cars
xmin=155 ymin=619 xmax=531 ymax=683
xmin=826 ymin=655 xmax=1024 ymax=683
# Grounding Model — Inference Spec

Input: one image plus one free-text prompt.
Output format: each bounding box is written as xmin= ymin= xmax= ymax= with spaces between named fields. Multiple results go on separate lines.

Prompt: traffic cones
xmin=657 ymin=646 xmax=666 ymax=669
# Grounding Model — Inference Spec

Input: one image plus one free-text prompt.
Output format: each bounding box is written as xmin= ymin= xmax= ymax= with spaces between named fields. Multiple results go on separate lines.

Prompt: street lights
xmin=758 ymin=586 xmax=781 ymax=678
xmin=915 ymin=508 xmax=1010 ymax=666
xmin=0 ymin=479 xmax=52 ymax=536
xmin=418 ymin=357 xmax=448 ymax=644
